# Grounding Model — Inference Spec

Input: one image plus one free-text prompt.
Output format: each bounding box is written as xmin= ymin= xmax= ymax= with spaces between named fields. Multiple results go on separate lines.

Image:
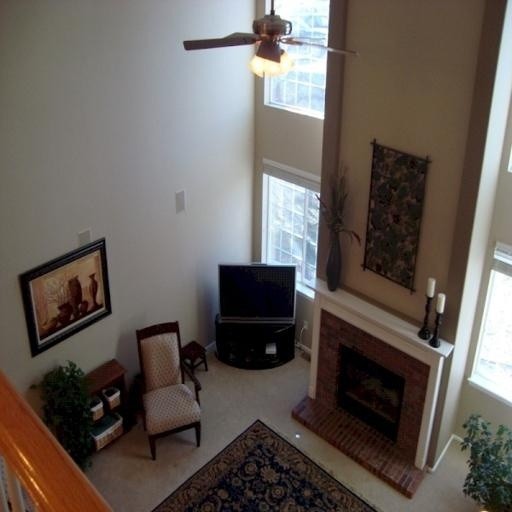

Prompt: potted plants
xmin=29 ymin=360 xmax=96 ymax=474
xmin=316 ymin=161 xmax=361 ymax=291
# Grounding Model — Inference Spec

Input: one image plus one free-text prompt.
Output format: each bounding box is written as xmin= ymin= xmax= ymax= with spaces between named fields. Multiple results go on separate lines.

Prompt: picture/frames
xmin=18 ymin=238 xmax=112 ymax=356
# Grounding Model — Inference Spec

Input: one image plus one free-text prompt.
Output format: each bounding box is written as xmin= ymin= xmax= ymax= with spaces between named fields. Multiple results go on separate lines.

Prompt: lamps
xmin=247 ymin=48 xmax=297 ymax=78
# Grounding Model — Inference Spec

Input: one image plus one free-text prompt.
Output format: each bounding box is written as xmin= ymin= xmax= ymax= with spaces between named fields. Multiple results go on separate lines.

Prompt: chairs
xmin=136 ymin=321 xmax=202 ymax=461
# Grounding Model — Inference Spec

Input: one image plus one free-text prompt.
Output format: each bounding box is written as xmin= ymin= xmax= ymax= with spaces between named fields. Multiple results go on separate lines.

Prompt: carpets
xmin=150 ymin=419 xmax=377 ymax=512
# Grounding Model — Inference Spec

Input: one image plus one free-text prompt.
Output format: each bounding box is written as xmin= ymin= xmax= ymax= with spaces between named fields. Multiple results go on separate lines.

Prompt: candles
xmin=427 ymin=278 xmax=446 ymax=314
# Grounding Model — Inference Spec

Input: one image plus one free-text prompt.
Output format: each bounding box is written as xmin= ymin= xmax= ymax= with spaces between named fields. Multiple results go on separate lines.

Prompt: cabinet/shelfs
xmin=214 ymin=314 xmax=297 ymax=370
xmin=57 ymin=359 xmax=137 ymax=459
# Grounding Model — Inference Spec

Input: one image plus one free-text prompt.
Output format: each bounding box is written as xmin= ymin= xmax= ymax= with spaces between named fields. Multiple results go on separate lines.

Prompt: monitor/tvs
xmin=218 ymin=264 xmax=297 ymax=325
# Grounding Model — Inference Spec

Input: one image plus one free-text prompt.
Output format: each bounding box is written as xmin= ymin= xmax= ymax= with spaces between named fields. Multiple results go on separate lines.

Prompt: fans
xmin=182 ymin=0 xmax=358 ymax=64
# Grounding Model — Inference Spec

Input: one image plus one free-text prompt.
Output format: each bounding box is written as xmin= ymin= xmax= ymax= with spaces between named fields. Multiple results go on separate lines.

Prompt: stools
xmin=182 ymin=341 xmax=208 ymax=375
